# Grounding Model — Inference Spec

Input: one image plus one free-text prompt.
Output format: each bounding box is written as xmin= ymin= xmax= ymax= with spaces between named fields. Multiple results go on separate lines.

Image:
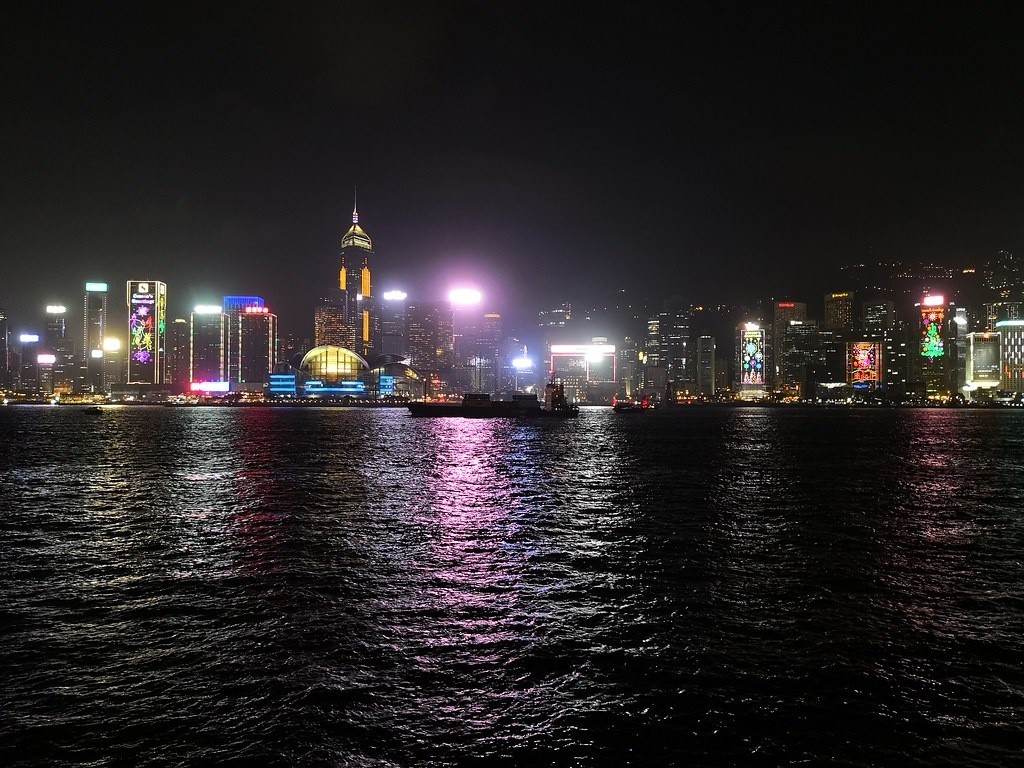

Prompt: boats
xmin=82 ymin=406 xmax=104 ymax=415
xmin=404 ymin=369 xmax=581 ymax=419
